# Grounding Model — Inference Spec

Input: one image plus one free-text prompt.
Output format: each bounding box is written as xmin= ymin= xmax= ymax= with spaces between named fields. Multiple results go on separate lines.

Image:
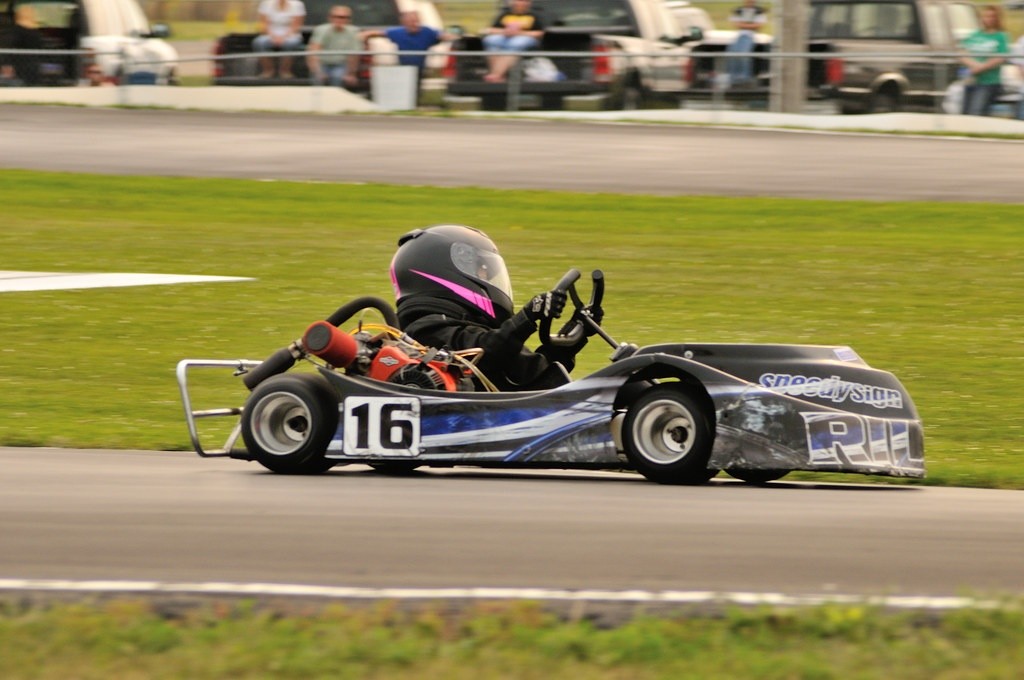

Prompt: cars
xmin=805 ymin=0 xmax=985 ymax=115
xmin=0 ymin=0 xmax=181 ymax=87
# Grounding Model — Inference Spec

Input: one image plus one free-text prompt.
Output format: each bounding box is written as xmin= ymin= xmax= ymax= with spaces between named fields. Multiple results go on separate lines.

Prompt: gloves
xmin=572 ymin=303 xmax=604 ymax=337
xmin=522 ymin=289 xmax=568 ymax=321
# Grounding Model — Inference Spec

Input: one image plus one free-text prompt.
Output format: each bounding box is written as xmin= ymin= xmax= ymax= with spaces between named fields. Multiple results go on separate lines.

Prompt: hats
xmin=140 ymin=21 xmax=172 ymax=39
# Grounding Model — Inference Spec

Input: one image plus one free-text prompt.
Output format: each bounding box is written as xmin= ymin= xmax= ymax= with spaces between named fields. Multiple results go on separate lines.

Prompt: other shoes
xmin=278 ymin=70 xmax=294 ymax=79
xmin=258 ymin=69 xmax=274 ymax=79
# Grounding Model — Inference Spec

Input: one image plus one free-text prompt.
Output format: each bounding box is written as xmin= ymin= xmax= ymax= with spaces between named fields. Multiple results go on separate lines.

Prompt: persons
xmin=305 ymin=5 xmax=364 ymax=86
xmin=954 ymin=5 xmax=1010 ymax=115
xmin=728 ymin=0 xmax=770 ymax=30
xmin=253 ymin=0 xmax=307 ymax=80
xmin=391 ymin=224 xmax=604 ymax=392
xmin=120 ymin=29 xmax=178 ymax=86
xmin=361 ymin=9 xmax=461 ymax=77
xmin=477 ymin=0 xmax=544 ymax=83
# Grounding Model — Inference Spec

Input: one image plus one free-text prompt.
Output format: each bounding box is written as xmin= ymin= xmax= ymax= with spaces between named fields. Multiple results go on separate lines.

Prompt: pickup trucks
xmin=421 ymin=0 xmax=702 ymax=111
xmin=213 ymin=0 xmax=462 ymax=93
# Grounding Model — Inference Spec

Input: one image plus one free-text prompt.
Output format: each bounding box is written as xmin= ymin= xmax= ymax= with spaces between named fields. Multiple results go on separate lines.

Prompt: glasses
xmin=329 ymin=15 xmax=349 ymax=20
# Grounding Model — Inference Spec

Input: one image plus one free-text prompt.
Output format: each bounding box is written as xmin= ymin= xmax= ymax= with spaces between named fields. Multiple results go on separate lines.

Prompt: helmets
xmin=390 ymin=223 xmax=515 ymax=329
